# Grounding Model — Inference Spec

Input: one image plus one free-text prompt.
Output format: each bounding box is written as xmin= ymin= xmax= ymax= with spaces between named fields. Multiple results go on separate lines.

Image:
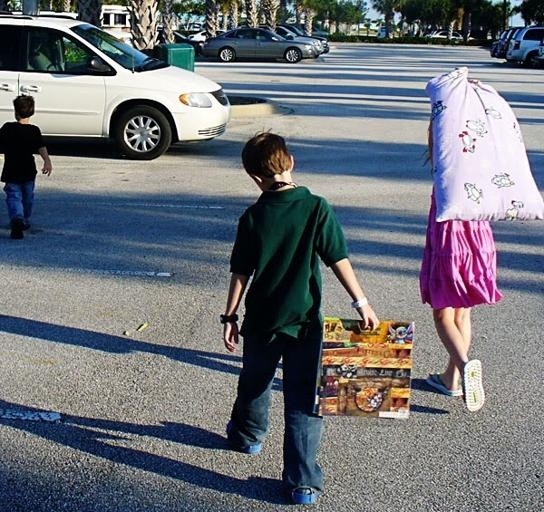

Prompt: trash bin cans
xmin=166 ymin=42 xmax=194 ymax=73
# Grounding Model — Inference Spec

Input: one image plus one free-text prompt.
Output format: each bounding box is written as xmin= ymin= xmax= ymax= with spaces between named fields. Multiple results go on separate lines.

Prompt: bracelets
xmin=351 ymin=296 xmax=368 ymax=309
xmin=220 ymin=313 xmax=239 ymax=324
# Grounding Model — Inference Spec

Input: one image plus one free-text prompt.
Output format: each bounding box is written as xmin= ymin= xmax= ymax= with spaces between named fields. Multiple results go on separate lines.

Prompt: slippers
xmin=463 ymin=359 xmax=486 ymax=411
xmin=427 ymin=371 xmax=461 ymax=400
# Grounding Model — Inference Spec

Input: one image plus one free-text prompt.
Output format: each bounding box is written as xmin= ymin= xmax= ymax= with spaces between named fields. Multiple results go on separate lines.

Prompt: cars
xmin=489 ymin=25 xmax=543 ymax=69
xmin=152 ymin=19 xmax=335 ymax=64
xmin=0 ymin=9 xmax=232 ymax=158
xmin=424 ymin=28 xmax=477 ymax=41
xmin=376 ymin=27 xmax=394 ymax=40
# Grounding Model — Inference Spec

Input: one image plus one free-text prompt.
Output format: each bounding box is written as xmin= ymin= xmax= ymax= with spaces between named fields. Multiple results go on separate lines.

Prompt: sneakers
xmin=286 ymin=479 xmax=320 ymax=504
xmin=9 ymin=216 xmax=25 ymax=242
xmin=227 ymin=421 xmax=263 ymax=454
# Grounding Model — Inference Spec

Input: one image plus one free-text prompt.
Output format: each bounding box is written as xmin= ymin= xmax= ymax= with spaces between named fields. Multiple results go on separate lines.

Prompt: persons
xmin=418 ymin=106 xmax=503 ymax=412
xmin=0 ymin=93 xmax=52 ymax=239
xmin=218 ymin=125 xmax=379 ymax=505
